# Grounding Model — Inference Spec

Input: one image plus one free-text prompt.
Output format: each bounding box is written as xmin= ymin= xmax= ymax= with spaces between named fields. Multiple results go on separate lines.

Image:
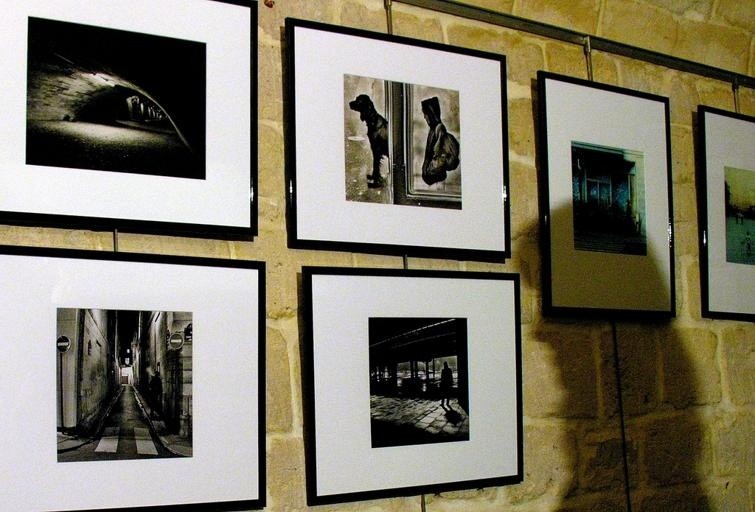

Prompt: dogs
xmin=349 ymin=93 xmax=389 ymax=181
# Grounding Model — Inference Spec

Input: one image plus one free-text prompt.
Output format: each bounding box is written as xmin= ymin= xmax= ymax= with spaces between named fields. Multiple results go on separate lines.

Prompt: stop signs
xmin=56 ymin=336 xmax=71 ymax=353
xmin=168 ymin=334 xmax=184 ymax=350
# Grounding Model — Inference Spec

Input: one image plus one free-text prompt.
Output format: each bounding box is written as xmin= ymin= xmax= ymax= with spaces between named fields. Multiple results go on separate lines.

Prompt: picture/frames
xmin=535 ymin=67 xmax=677 ymax=321
xmin=696 ymin=101 xmax=754 ymax=323
xmin=0 ymin=243 xmax=268 ymax=512
xmin=299 ymin=264 xmax=524 ymax=506
xmin=0 ymin=0 xmax=260 ymax=238
xmin=283 ymin=16 xmax=513 ymax=261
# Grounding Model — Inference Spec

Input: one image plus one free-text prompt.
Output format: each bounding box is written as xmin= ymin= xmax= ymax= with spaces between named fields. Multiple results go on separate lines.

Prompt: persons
xmin=420 ymin=97 xmax=459 ymax=191
xmin=149 ymin=371 xmax=162 ymax=416
xmin=440 ymin=360 xmax=454 ymax=408
